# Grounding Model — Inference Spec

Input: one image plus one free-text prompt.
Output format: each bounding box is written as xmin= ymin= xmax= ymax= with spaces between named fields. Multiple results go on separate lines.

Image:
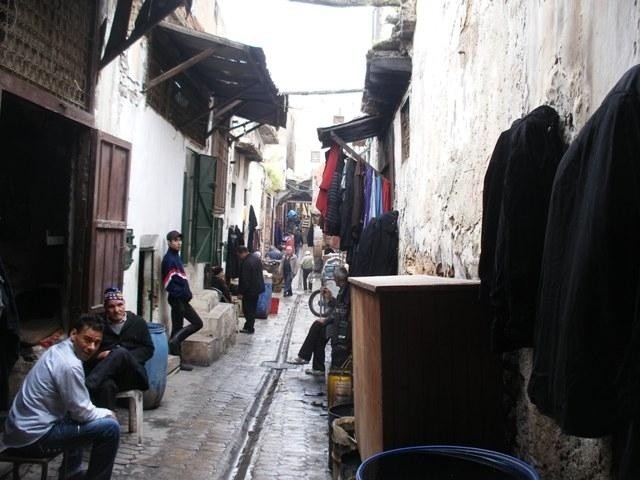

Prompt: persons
xmin=265 ymin=245 xmax=281 ymax=259
xmin=0 ymin=264 xmax=22 ymax=411
xmin=0 ymin=314 xmax=120 ymax=479
xmin=321 ymin=244 xmax=332 ymax=261
xmin=278 ymin=246 xmax=297 ymax=296
xmin=209 ymin=264 xmax=232 ymax=302
xmin=161 ymin=230 xmax=203 ymax=370
xmin=300 ymin=250 xmax=315 ymax=291
xmin=83 ymin=288 xmax=154 ymax=411
xmin=272 ymin=214 xmax=304 ymax=258
xmin=236 ymin=246 xmax=264 ymax=334
xmin=292 ymin=264 xmax=349 ymax=375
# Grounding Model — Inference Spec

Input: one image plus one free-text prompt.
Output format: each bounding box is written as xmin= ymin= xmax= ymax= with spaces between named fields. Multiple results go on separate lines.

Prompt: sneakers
xmin=305 ymin=368 xmax=325 ymax=376
xmin=287 ymin=357 xmax=309 ymax=365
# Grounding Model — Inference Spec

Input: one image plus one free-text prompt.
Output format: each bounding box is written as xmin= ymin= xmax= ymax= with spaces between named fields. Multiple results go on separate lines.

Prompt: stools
xmin=0 ymin=447 xmax=66 ymax=479
xmin=117 ymin=389 xmax=144 ymax=433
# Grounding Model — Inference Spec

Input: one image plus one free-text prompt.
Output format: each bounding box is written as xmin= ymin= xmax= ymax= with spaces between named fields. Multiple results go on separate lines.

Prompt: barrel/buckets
xmin=327 ymin=356 xmax=353 ymax=406
xmin=355 ymin=445 xmax=540 ymax=480
xmin=116 ymin=322 xmax=169 ymax=410
xmin=329 ymin=403 xmax=356 ymax=471
xmin=332 ymin=417 xmax=357 ymax=479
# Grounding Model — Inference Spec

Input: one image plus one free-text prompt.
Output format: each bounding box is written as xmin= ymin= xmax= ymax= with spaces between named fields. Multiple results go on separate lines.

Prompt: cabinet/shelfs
xmin=348 ymin=272 xmax=510 ymax=464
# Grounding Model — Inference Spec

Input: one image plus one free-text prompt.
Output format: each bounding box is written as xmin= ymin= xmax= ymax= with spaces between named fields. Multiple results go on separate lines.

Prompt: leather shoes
xmin=239 ymin=328 xmax=255 ymax=334
xmin=180 ymin=364 xmax=194 ymax=371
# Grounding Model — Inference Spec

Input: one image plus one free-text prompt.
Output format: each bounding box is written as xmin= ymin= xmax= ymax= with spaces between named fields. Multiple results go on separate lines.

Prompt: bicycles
xmin=309 ymin=270 xmax=336 ymax=318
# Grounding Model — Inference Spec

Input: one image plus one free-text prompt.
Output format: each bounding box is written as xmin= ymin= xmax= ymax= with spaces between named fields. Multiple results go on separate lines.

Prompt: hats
xmin=167 ymin=230 xmax=183 ymax=241
xmin=104 ymin=286 xmax=124 ymax=302
